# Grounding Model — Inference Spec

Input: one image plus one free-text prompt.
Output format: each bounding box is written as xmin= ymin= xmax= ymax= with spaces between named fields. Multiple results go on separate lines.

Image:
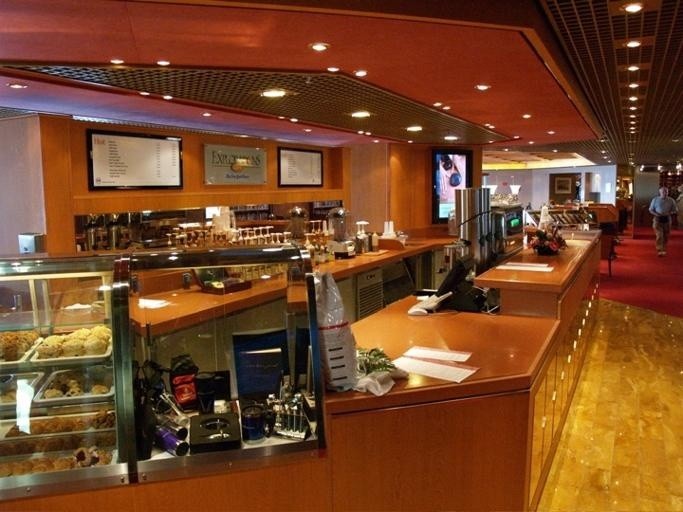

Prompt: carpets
xmin=598 ymin=224 xmax=682 ymax=320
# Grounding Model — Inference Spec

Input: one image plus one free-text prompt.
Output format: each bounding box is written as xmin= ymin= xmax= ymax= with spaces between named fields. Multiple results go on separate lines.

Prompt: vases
xmin=538 ymin=247 xmax=558 ymax=256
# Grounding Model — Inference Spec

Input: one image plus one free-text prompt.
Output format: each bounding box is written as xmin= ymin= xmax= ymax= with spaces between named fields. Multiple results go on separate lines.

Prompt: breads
xmin=0 ymin=325 xmax=115 ymax=478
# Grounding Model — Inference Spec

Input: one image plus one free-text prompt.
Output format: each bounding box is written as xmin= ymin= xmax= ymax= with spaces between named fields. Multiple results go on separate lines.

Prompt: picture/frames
xmin=555 ymin=178 xmax=572 ymax=194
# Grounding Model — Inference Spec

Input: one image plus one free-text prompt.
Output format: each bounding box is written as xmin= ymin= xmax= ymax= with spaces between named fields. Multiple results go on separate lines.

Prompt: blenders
xmin=329 ymin=207 xmax=359 ymax=258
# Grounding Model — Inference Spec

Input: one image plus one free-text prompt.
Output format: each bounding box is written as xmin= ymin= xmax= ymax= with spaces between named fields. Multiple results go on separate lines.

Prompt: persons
xmin=648 ymin=186 xmax=677 ymax=256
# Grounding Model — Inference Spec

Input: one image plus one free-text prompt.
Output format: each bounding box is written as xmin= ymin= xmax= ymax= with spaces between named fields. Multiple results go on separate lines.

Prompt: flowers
xmin=530 ymin=220 xmax=567 ymax=252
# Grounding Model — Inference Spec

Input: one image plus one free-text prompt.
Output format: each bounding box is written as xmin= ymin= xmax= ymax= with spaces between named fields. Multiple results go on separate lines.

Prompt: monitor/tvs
xmin=435 ymin=255 xmax=475 ymax=297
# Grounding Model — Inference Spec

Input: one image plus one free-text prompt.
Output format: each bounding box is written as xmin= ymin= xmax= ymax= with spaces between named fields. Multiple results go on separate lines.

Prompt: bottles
xmin=152 ymin=372 xmax=214 ymax=456
xmin=358 ymin=222 xmax=379 ymax=254
xmin=305 ymin=220 xmax=336 ymax=266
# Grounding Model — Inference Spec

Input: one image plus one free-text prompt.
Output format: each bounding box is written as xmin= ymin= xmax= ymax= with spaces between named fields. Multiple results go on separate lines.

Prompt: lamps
xmin=508 ymin=184 xmax=521 ymax=202
xmin=483 ymin=185 xmax=497 ymax=198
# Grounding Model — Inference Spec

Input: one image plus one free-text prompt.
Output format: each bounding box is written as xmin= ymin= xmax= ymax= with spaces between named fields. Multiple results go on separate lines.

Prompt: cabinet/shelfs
xmin=0 ymin=242 xmax=330 ymax=501
xmin=136 ymin=298 xmax=286 ymax=372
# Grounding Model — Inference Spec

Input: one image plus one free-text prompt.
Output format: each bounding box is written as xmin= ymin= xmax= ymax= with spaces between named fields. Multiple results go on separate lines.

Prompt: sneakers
xmin=658 ymin=250 xmax=666 ymax=255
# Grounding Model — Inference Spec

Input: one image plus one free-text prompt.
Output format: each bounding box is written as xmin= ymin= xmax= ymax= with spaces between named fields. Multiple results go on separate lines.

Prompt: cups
xmin=241 ymin=406 xmax=274 ymax=444
